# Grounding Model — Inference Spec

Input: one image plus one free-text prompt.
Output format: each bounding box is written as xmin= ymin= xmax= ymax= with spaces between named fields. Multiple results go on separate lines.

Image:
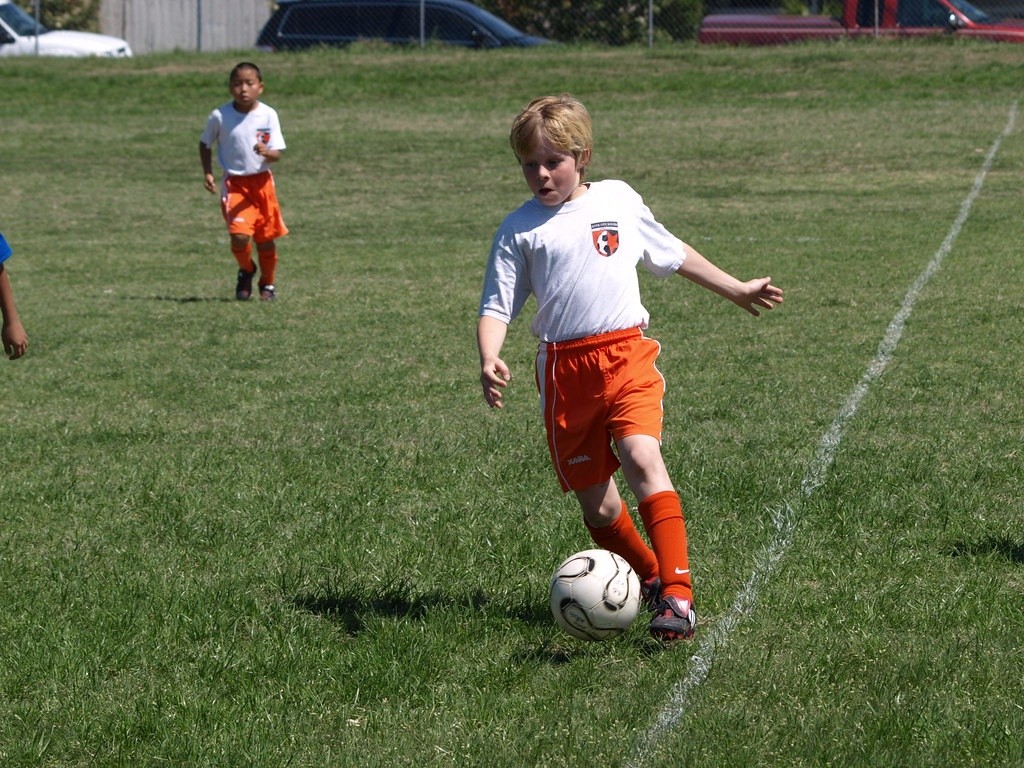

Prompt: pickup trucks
xmin=696 ymin=0 xmax=1024 ymax=45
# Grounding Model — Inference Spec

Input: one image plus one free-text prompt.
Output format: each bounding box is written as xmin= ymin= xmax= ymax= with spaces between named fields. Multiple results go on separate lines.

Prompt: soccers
xmin=549 ymin=548 xmax=643 ymax=641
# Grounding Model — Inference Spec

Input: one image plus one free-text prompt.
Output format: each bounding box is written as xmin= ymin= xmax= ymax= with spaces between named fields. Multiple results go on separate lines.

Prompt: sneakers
xmin=642 ymin=596 xmax=698 ymax=639
xmin=640 ymin=576 xmax=662 ymax=612
xmin=236 ymin=258 xmax=257 ymax=301
xmin=258 ymin=282 xmax=275 ymax=300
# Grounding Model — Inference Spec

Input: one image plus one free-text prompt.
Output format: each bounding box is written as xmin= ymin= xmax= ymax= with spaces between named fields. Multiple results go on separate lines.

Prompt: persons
xmin=199 ymin=62 xmax=287 ymax=301
xmin=477 ymin=94 xmax=782 ymax=640
xmin=0 ymin=232 xmax=30 ymax=360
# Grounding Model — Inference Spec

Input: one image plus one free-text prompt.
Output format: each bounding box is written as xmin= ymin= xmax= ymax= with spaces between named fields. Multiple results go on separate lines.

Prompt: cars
xmin=0 ymin=0 xmax=134 ymax=59
xmin=251 ymin=0 xmax=572 ymax=56
